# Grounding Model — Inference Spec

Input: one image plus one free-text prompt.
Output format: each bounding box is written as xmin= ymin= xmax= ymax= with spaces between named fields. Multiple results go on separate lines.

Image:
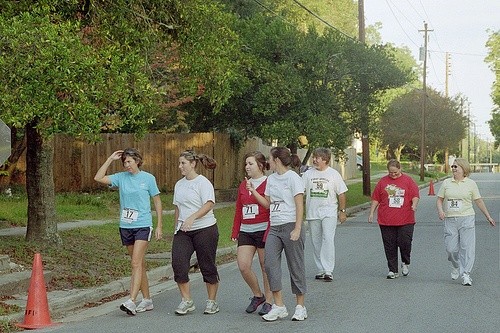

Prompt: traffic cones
xmin=13 ymin=253 xmax=64 ymax=329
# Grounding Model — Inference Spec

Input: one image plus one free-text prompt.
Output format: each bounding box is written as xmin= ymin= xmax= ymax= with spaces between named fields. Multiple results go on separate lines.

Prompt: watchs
xmin=340 ymin=209 xmax=346 ymax=212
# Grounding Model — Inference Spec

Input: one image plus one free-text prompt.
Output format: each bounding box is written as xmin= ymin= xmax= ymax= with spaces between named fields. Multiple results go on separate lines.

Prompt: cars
xmin=356 ymin=155 xmax=372 ymax=172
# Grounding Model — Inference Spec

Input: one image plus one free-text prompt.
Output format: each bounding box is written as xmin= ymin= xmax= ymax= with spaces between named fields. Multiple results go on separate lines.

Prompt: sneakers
xmin=461 ymin=272 xmax=472 ymax=286
xmin=291 ymin=304 xmax=307 ymax=321
xmin=120 ymin=299 xmax=137 ymax=316
xmin=450 ymin=267 xmax=460 ymax=280
xmin=400 ymin=262 xmax=410 ymax=277
xmin=386 ymin=271 xmax=399 ymax=279
xmin=135 ymin=298 xmax=154 ymax=313
xmin=262 ymin=304 xmax=288 ymax=321
xmin=258 ymin=303 xmax=272 ymax=315
xmin=246 ymin=293 xmax=266 ymax=313
xmin=174 ymin=297 xmax=196 ymax=315
xmin=203 ymin=300 xmax=219 ymax=314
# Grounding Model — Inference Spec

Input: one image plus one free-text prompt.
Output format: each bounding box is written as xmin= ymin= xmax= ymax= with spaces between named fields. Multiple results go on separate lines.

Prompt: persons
xmin=368 ymin=160 xmax=420 ymax=279
xmin=245 ymin=147 xmax=308 ymax=321
xmin=231 ymin=152 xmax=272 ymax=314
xmin=172 ymin=150 xmax=220 ymax=314
xmin=300 ymin=147 xmax=347 ymax=281
xmin=437 ymin=159 xmax=495 ymax=285
xmin=94 ymin=148 xmax=163 ymax=316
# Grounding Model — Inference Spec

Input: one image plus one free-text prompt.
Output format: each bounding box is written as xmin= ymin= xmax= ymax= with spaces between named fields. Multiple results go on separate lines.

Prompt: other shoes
xmin=315 ymin=273 xmax=334 ymax=280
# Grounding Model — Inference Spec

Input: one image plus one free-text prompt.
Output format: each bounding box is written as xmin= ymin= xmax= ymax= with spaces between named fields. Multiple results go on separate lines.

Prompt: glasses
xmin=123 ymin=150 xmax=136 ymax=157
xmin=451 ymin=165 xmax=459 ymax=168
